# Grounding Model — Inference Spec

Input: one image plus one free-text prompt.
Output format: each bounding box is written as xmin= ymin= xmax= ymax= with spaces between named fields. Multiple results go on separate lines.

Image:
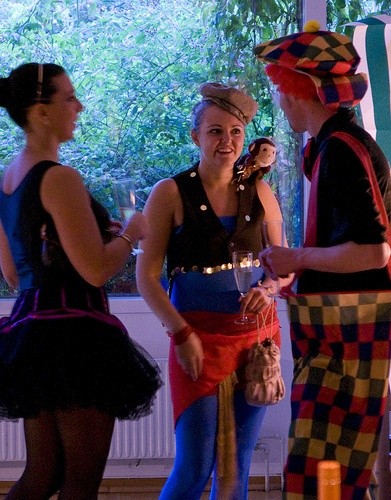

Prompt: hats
xmin=252 ymin=30 xmax=368 ymax=113
xmin=199 ymin=80 xmax=258 ymax=128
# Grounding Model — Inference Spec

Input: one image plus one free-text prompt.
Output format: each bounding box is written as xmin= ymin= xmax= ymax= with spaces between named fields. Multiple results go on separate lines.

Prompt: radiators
xmin=0 ymin=356 xmax=178 ymax=462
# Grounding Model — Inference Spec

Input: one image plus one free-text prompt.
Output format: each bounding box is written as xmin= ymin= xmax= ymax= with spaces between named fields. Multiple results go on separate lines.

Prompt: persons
xmin=135 ymin=80 xmax=295 ymax=500
xmin=250 ymin=22 xmax=391 ymax=500
xmin=0 ymin=63 xmax=166 ymax=500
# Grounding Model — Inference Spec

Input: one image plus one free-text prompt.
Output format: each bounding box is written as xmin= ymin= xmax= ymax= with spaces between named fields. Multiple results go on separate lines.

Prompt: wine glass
xmin=263 ymin=220 xmax=288 ymax=298
xmin=113 ymin=177 xmax=144 ymax=255
xmin=231 ymin=250 xmax=256 ymax=324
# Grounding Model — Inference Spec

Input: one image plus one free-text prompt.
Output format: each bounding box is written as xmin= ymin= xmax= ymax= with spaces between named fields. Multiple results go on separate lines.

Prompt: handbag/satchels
xmin=243 ymin=297 xmax=286 ymax=407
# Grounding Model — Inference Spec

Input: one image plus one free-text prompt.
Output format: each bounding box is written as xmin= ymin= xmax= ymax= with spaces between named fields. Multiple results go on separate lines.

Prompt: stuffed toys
xmin=233 ymin=138 xmax=277 ymax=184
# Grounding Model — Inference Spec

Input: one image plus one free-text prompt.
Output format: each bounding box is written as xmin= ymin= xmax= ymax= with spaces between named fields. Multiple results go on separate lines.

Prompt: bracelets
xmin=171 ymin=326 xmax=191 ymax=345
xmin=119 ymin=232 xmax=134 ymax=252
